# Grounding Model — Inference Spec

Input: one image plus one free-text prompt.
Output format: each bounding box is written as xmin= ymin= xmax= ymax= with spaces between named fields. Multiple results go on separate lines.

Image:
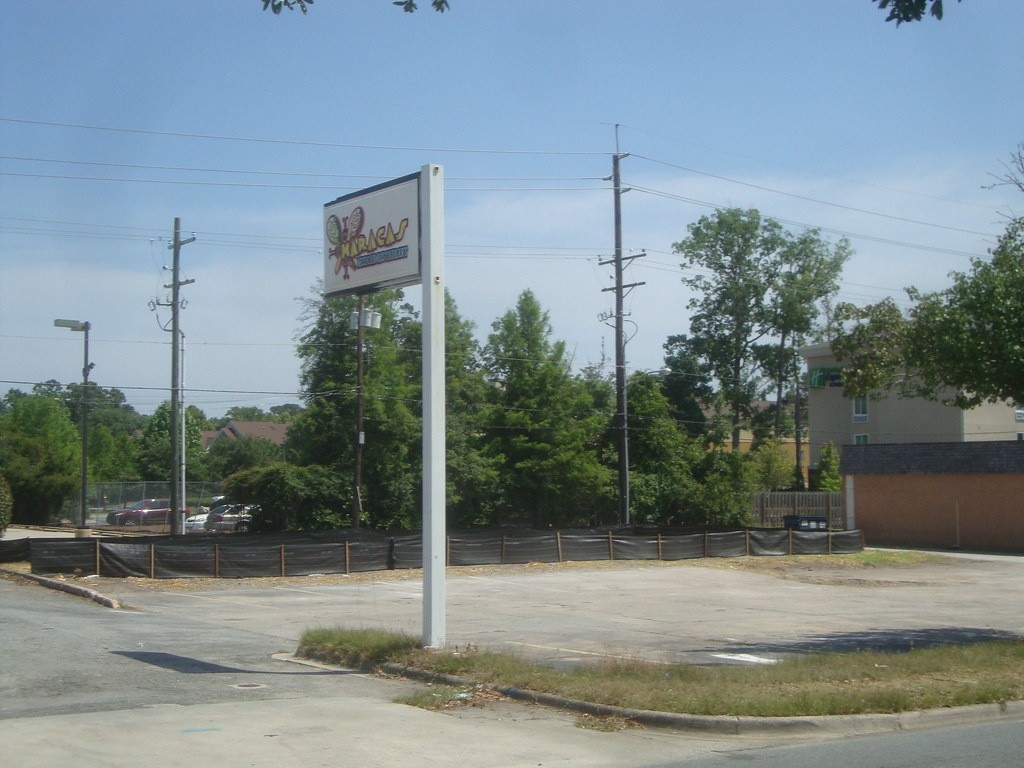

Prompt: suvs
xmin=106 ymin=499 xmax=189 ymax=526
xmin=185 ymin=504 xmax=264 ymax=534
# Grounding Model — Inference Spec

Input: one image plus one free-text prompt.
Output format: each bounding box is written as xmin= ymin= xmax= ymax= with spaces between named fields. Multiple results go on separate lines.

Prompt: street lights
xmin=616 ymin=368 xmax=672 ymax=523
xmin=55 ymin=319 xmax=92 ymax=529
